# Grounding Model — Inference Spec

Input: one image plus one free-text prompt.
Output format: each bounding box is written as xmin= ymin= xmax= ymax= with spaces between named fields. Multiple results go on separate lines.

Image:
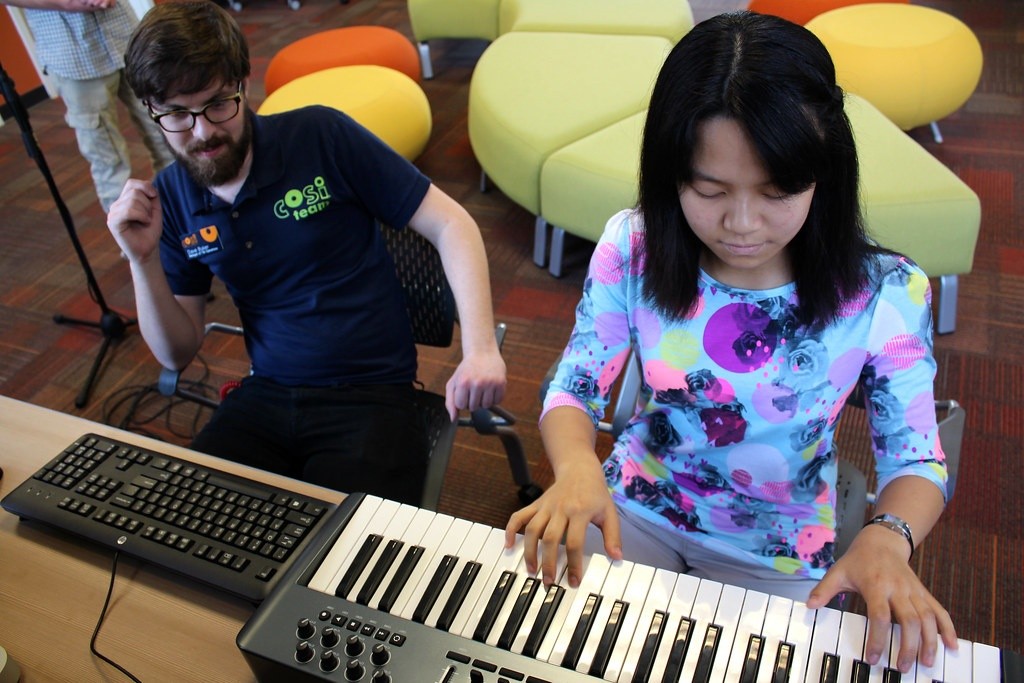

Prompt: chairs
xmin=157 ymin=221 xmax=545 ymax=513
xmin=538 ymin=350 xmax=964 ymax=612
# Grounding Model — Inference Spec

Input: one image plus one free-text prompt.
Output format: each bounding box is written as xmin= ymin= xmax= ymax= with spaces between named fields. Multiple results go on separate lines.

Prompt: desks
xmin=0 ymin=396 xmax=348 ymax=683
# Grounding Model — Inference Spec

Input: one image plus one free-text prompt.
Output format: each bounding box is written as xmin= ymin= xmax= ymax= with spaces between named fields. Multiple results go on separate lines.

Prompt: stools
xmin=539 ymin=109 xmax=641 ymax=278
xmin=257 ymin=65 xmax=433 ymax=164
xmin=407 ymin=0 xmax=500 ymax=82
xmin=466 ymin=31 xmax=676 ymax=269
xmin=496 ymin=0 xmax=695 ymax=46
xmin=748 ymin=0 xmax=908 ymax=27
xmin=803 ymin=3 xmax=983 ymax=144
xmin=844 ymin=93 xmax=983 ymax=336
xmin=264 ymin=26 xmax=422 ymax=97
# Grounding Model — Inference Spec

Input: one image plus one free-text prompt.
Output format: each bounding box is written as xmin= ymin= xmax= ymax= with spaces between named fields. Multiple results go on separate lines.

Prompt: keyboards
xmin=0 ymin=433 xmax=337 ymax=602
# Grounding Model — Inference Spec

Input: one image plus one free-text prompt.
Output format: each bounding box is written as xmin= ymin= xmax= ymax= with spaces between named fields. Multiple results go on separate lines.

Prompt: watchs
xmin=860 ymin=513 xmax=915 ymax=562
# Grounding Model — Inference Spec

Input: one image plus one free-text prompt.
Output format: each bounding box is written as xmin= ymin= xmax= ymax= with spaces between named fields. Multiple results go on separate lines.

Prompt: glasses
xmin=144 ymin=80 xmax=243 ymax=133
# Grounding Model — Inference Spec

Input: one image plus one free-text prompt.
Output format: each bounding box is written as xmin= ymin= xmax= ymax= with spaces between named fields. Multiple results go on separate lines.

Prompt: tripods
xmin=0 ymin=67 xmax=140 ymax=409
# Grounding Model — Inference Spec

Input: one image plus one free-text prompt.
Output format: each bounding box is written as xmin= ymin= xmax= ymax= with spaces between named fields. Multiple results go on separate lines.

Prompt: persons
xmin=505 ymin=12 xmax=959 ymax=672
xmin=0 ymin=0 xmax=508 ymax=509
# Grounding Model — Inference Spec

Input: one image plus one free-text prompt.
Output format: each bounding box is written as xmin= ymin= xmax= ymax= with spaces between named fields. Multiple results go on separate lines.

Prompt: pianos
xmin=231 ymin=473 xmax=1018 ymax=683
